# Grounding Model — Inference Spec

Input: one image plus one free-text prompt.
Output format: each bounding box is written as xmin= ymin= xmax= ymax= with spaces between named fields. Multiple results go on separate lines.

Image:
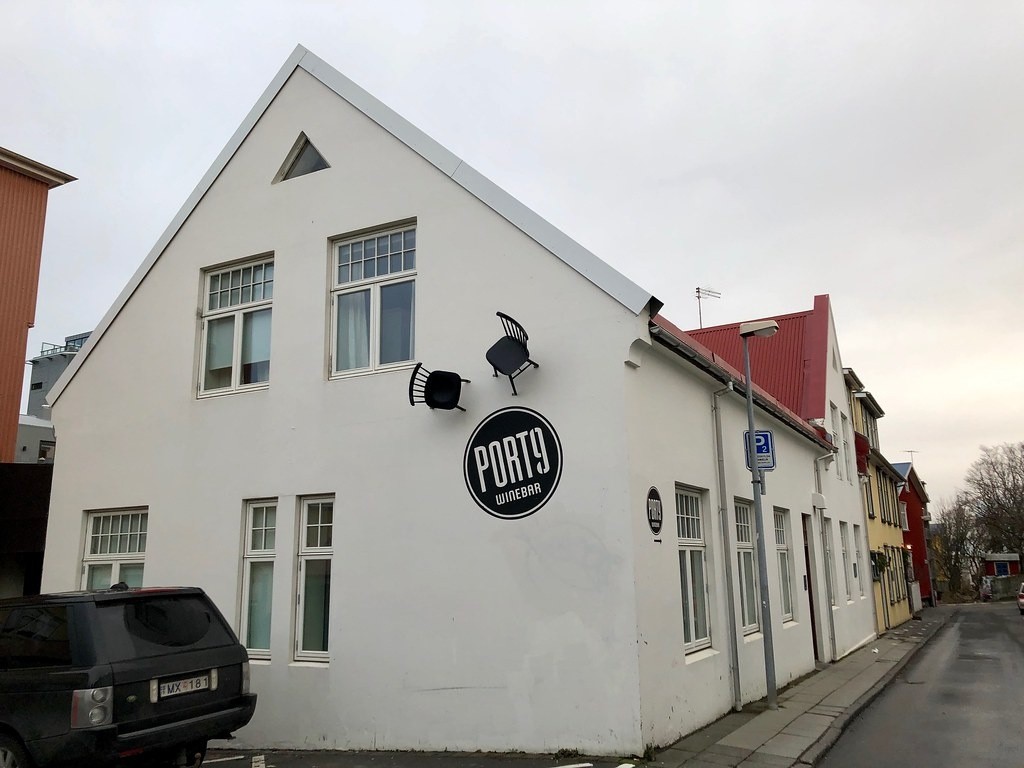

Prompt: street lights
xmin=738 ymin=320 xmax=782 ymax=712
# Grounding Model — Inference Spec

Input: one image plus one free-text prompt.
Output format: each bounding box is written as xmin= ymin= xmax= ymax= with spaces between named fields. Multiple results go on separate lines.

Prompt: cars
xmin=1016 ymin=582 xmax=1024 ymax=612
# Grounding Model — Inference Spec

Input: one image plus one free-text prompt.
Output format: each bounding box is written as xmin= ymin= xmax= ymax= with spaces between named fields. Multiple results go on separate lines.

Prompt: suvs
xmin=1 ymin=582 xmax=260 ymax=768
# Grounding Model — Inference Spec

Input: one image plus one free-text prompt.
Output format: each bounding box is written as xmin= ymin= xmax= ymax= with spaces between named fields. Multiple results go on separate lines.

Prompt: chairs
xmin=485 ymin=311 xmax=539 ymax=397
xmin=408 ymin=362 xmax=472 ymax=413
